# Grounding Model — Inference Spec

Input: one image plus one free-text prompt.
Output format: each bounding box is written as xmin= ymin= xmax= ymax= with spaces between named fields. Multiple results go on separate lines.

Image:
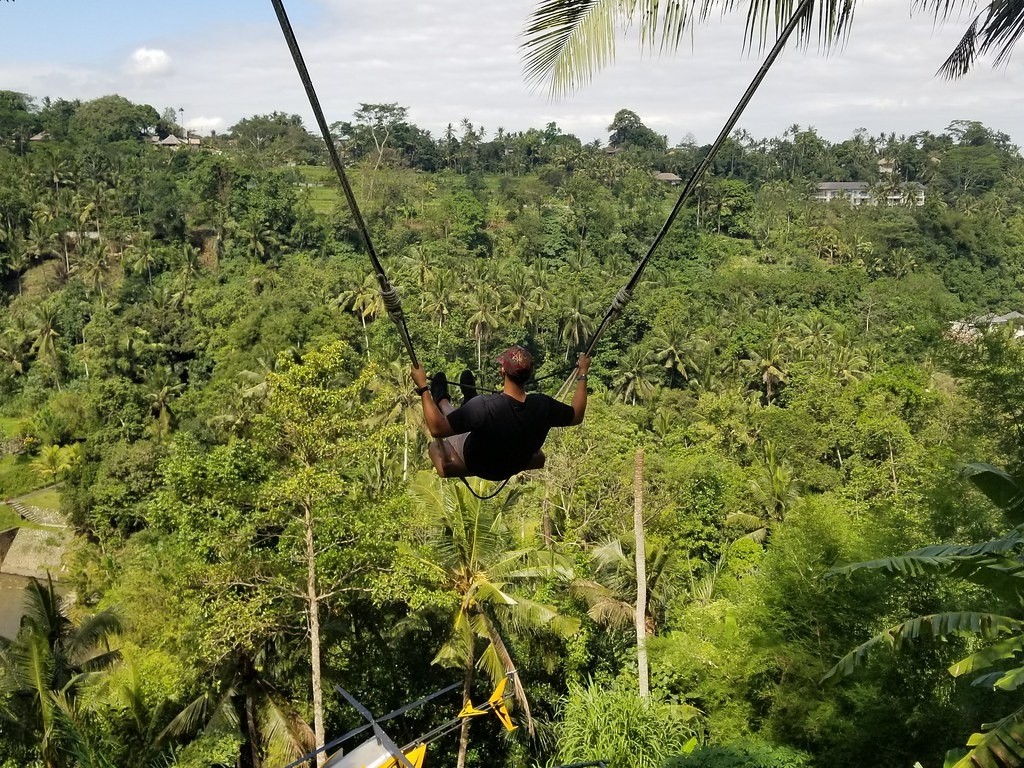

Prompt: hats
xmin=497 ymin=345 xmax=533 ymax=380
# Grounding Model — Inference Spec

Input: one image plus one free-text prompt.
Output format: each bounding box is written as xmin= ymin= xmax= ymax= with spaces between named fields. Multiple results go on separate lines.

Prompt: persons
xmin=411 ymin=345 xmax=588 ymax=480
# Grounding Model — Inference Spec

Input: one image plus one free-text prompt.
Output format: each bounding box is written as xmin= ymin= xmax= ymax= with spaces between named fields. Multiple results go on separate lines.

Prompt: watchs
xmin=414 ymin=383 xmax=431 ymax=396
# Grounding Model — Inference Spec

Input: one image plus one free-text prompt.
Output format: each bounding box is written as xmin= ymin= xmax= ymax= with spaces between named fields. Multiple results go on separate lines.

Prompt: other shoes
xmin=431 ymin=372 xmax=451 ymax=408
xmin=459 ymin=370 xmax=478 ymax=405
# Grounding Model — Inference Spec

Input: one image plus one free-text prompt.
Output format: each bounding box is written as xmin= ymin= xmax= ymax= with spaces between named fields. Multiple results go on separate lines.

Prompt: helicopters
xmin=278 ymin=665 xmax=518 ymax=768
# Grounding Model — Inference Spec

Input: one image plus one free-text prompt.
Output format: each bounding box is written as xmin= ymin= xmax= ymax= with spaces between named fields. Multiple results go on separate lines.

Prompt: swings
xmin=272 ymin=0 xmax=813 ymax=478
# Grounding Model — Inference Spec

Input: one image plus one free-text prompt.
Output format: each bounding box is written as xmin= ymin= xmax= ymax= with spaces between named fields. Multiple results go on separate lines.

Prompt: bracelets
xmin=575 ymin=373 xmax=587 ymax=382
